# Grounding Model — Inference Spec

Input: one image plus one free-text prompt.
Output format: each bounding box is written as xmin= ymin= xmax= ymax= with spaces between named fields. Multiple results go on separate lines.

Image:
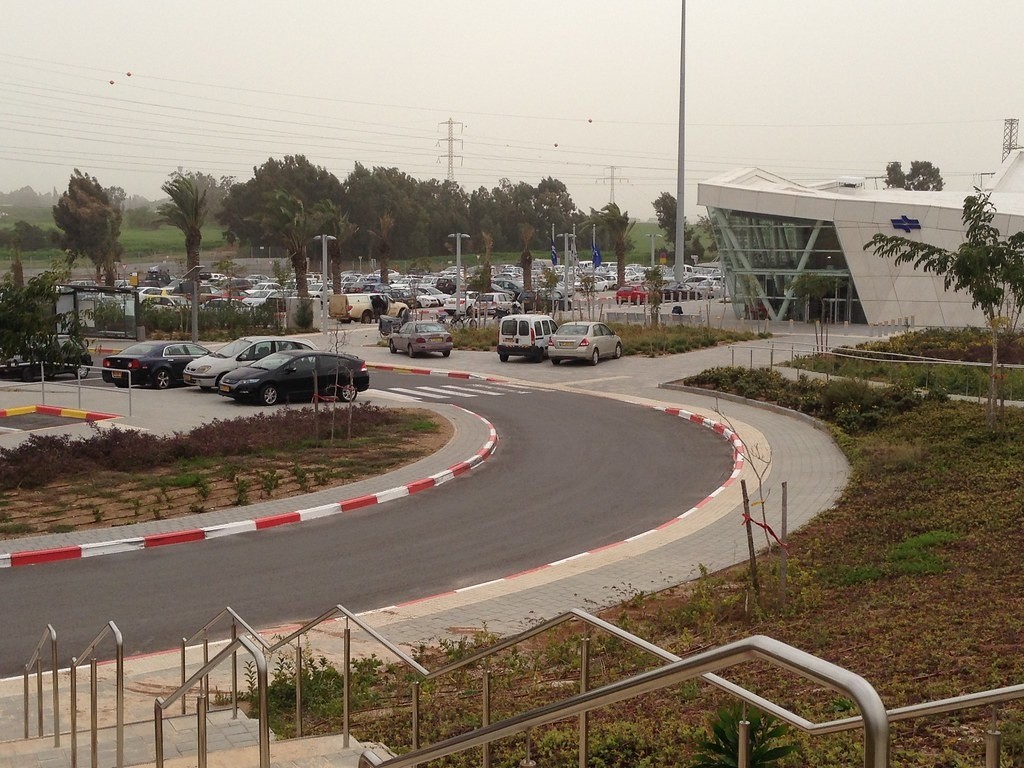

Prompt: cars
xmin=443 ymin=291 xmax=479 ymax=316
xmin=102 ymin=340 xmax=214 ymax=390
xmin=389 ymin=321 xmax=453 ymax=358
xmin=695 ymin=280 xmax=725 ymax=300
xmin=0 ymin=334 xmax=95 ymax=383
xmin=473 ymin=292 xmax=521 ymax=319
xmin=661 ymin=283 xmax=701 ymax=302
xmin=50 ymin=258 xmax=725 ymax=313
xmin=615 ymin=285 xmax=660 ymax=305
xmin=547 ymin=321 xmax=623 ymax=366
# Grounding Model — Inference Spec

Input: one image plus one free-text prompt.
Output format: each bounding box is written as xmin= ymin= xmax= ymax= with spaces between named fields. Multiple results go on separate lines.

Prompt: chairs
xmin=255 ymin=347 xmax=269 ymax=359
xmin=170 ymin=348 xmax=182 ymax=354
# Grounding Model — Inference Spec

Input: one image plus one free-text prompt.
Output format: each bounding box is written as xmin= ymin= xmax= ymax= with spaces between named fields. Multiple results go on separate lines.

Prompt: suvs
xmin=218 ymin=349 xmax=370 ymax=406
xmin=183 ymin=335 xmax=320 ymax=393
xmin=517 ymin=289 xmax=572 ymax=314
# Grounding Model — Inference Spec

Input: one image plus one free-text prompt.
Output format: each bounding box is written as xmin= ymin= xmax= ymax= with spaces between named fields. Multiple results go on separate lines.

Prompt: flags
xmin=592 ymin=242 xmax=602 ymax=267
xmin=551 ymin=239 xmax=558 ymax=265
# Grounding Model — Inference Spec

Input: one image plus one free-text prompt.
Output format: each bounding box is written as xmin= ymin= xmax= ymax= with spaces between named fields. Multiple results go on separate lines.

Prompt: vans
xmin=497 ymin=315 xmax=559 ymax=363
xmin=328 ymin=293 xmax=407 ymax=324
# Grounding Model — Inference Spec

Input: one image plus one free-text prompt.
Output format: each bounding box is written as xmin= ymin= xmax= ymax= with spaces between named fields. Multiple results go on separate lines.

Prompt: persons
xmin=390 ymin=280 xmax=394 ymax=283
xmin=373 ymin=296 xmax=386 ymax=323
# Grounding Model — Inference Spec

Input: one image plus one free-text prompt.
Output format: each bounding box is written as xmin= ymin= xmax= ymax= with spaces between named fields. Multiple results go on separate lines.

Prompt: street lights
xmin=556 ymin=233 xmax=577 ymax=312
xmin=313 ymin=234 xmax=337 ymax=335
xmin=644 ymin=233 xmax=663 ymax=269
xmin=448 ymin=232 xmax=470 ymax=316
